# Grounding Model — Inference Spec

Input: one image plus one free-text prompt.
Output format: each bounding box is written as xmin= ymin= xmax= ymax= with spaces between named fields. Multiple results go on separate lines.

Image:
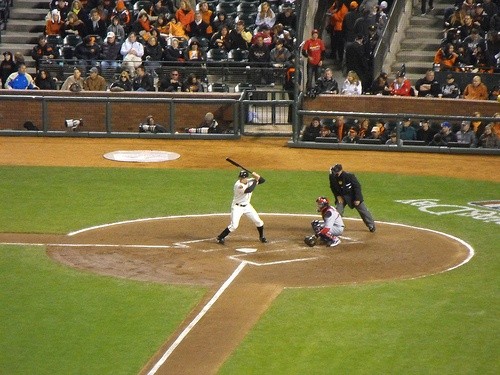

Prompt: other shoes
xmin=259 ymin=235 xmax=268 ymax=242
xmin=369 ymin=224 xmax=375 ymax=232
xmin=216 ymin=235 xmax=224 ymax=245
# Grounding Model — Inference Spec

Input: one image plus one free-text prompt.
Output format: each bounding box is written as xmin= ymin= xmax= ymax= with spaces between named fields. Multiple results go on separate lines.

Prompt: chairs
xmin=0 ymin=0 xmax=296 ymax=76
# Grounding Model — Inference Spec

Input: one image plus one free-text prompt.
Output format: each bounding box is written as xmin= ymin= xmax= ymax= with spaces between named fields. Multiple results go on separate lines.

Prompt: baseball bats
xmin=226 ymin=157 xmax=253 ymax=174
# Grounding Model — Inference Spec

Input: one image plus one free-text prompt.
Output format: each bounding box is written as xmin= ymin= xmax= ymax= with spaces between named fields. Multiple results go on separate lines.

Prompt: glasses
xmin=172 ymin=74 xmax=177 ymax=76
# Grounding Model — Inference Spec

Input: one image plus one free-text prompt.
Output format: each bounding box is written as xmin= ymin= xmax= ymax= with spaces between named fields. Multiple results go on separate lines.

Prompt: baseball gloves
xmin=304 ymin=235 xmax=316 ymax=247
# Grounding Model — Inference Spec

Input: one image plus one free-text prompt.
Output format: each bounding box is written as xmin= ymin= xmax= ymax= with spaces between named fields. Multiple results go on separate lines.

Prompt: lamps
xmin=64 ymin=118 xmax=84 ymax=133
xmin=184 ymin=126 xmax=212 ymax=134
xmin=138 ymin=123 xmax=158 ymax=134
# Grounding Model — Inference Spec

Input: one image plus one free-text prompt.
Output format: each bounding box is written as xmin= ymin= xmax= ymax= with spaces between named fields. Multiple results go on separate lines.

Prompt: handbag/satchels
xmin=326 ymin=24 xmax=334 ymax=34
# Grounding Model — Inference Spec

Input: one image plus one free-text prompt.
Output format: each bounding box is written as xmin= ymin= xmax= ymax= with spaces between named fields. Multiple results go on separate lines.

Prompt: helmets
xmin=395 ymin=71 xmax=405 ymax=78
xmin=316 ymin=196 xmax=330 ymax=211
xmin=239 ymin=171 xmax=248 ymax=177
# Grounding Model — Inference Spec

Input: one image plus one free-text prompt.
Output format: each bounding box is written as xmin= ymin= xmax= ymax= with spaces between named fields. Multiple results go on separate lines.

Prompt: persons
xmin=215 ymin=169 xmax=269 ymax=245
xmin=328 ymin=162 xmax=376 ymax=233
xmin=303 ymin=196 xmax=346 ymax=248
xmin=139 ymin=114 xmax=168 ymax=133
xmin=0 ymin=0 xmax=500 ymax=101
xmin=24 ymin=122 xmax=38 ymax=131
xmin=301 ymin=110 xmax=500 ymax=148
xmin=198 ymin=112 xmax=223 ymax=134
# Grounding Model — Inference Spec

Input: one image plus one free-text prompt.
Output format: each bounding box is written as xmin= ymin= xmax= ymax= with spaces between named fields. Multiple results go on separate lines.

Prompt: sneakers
xmin=330 ymin=237 xmax=342 ymax=247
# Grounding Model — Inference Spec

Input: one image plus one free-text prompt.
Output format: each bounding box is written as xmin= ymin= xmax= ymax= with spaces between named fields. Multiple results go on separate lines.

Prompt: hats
xmin=116 ymin=0 xmax=124 ymax=10
xmin=369 ymin=24 xmax=378 ymax=31
xmin=371 ymin=126 xmax=380 ymax=132
xmin=380 ymin=72 xmax=387 ymax=80
xmin=107 ymin=32 xmax=115 ymax=38
xmin=388 ymin=118 xmax=470 ymax=137
xmin=312 ymin=29 xmax=319 ymax=33
xmin=89 ymin=67 xmax=98 ymax=73
xmin=320 ymin=126 xmax=328 ymax=131
xmin=446 ymin=73 xmax=454 ymax=79
xmin=426 ymin=70 xmax=434 ymax=76
xmin=120 ymin=71 xmax=129 ymax=79
xmin=51 ymin=9 xmax=61 ymax=23
xmin=476 ymin=43 xmax=486 ymax=49
xmin=333 ymin=164 xmax=343 ymax=172
xmin=3 ymin=51 xmax=11 ymax=56
